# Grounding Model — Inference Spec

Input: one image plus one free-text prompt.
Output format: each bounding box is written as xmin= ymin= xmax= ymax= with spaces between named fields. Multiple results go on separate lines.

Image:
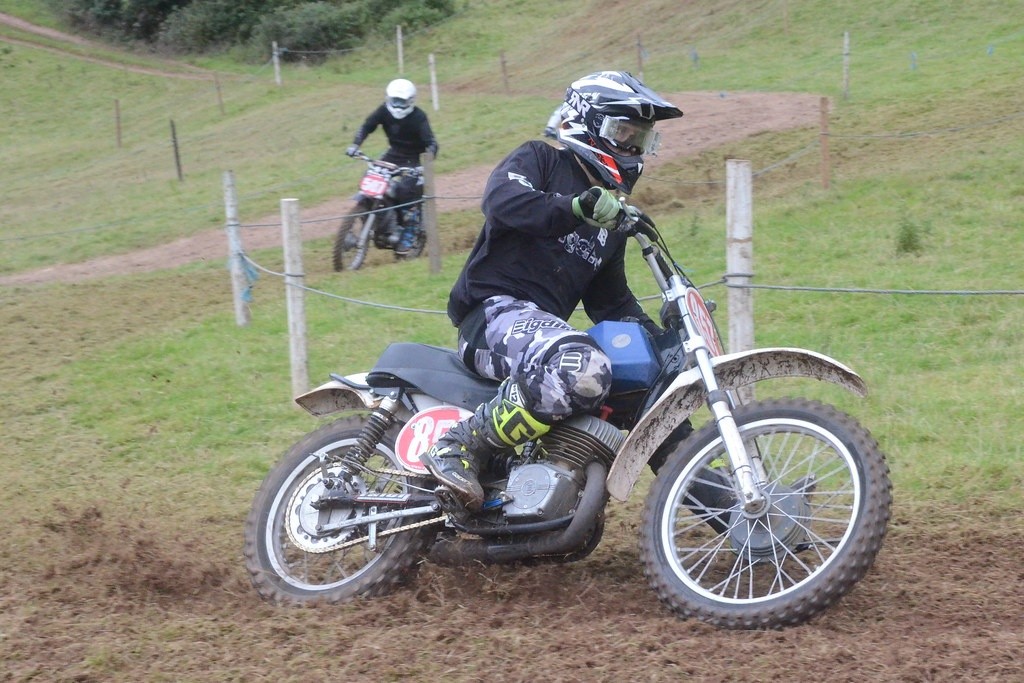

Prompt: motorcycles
xmin=332 ymin=148 xmax=428 ymax=272
xmin=238 ymin=190 xmax=900 ymax=633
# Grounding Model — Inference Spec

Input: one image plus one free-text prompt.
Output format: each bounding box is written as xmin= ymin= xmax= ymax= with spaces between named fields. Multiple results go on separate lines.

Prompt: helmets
xmin=544 ymin=71 xmax=683 ymax=195
xmin=384 ymin=79 xmax=416 ymax=119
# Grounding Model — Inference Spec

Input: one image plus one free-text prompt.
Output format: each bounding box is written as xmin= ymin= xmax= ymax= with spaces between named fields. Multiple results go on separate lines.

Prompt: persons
xmin=418 ymin=71 xmax=817 ymax=515
xmin=346 ymin=79 xmax=439 ymax=258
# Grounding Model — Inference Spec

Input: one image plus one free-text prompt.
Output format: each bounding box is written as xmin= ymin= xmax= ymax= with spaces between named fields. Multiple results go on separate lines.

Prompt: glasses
xmin=565 ymin=87 xmax=662 ymax=157
xmin=385 ymin=96 xmax=416 ymax=109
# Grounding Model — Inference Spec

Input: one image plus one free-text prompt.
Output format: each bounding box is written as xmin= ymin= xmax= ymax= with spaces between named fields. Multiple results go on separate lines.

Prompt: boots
xmin=418 ymin=377 xmax=550 ymax=513
xmin=396 ymin=205 xmax=420 ymax=255
xmin=650 ymin=437 xmax=816 ymax=540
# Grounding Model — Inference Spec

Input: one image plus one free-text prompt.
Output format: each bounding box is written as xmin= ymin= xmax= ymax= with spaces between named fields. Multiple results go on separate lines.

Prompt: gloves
xmin=348 ymin=145 xmax=362 ymax=156
xmin=572 ymin=186 xmax=620 ymax=229
xmin=412 ymin=165 xmax=424 ymax=175
